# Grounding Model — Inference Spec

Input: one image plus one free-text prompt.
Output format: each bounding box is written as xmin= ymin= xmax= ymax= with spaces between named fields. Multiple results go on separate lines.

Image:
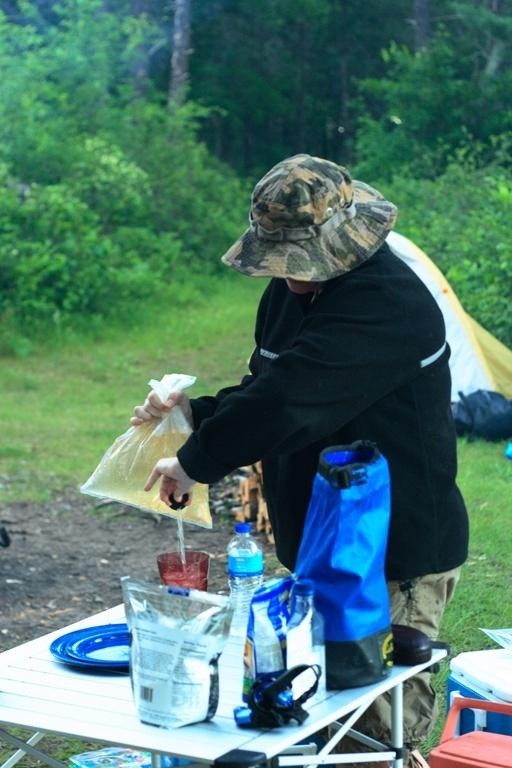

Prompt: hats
xmin=220 ymin=153 xmax=399 ymax=284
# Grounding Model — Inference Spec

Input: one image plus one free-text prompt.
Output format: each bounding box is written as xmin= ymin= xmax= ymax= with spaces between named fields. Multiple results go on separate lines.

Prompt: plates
xmin=49 ymin=623 xmax=129 ymax=668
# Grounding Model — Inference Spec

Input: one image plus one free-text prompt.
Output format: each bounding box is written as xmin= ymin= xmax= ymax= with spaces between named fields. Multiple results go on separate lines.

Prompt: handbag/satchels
xmin=453 ymin=390 xmax=511 ymax=442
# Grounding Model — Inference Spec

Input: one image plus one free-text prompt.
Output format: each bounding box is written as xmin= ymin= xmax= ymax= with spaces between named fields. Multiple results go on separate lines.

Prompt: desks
xmin=0 ymin=604 xmax=448 ymax=768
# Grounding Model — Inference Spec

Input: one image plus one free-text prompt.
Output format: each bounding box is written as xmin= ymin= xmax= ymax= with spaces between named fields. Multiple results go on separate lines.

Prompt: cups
xmin=156 ymin=550 xmax=210 ymax=593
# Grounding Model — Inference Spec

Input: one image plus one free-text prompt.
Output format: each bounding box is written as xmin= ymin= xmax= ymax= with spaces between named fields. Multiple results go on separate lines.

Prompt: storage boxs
xmin=448 ymin=649 xmax=512 ymax=734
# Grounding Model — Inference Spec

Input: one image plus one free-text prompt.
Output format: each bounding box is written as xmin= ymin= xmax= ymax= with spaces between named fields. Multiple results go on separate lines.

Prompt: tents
xmin=385 ymin=230 xmax=512 ymax=397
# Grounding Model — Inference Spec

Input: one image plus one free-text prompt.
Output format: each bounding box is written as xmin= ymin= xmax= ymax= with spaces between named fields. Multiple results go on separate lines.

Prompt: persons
xmin=130 ymin=153 xmax=470 ymax=768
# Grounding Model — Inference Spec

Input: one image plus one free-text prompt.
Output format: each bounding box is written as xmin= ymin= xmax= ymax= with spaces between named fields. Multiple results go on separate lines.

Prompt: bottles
xmin=285 ymin=578 xmax=327 ymax=707
xmin=226 ymin=523 xmax=264 ymax=633
